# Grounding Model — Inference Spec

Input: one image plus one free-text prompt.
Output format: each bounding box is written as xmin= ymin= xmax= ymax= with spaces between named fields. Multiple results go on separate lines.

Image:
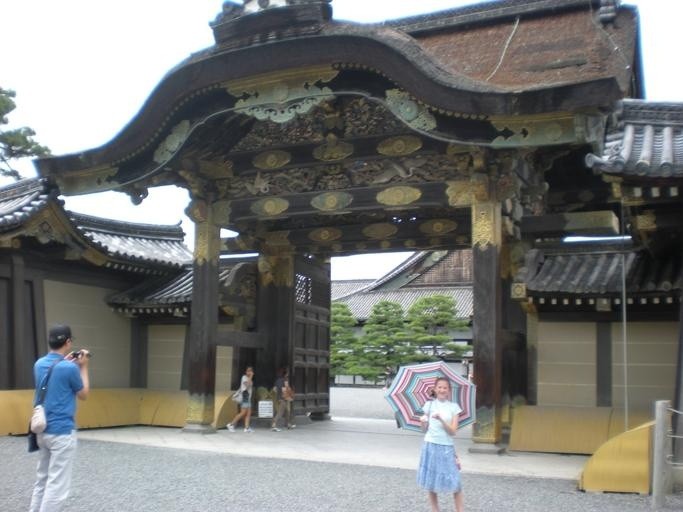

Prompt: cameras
xmin=71 ymin=351 xmax=83 ymax=360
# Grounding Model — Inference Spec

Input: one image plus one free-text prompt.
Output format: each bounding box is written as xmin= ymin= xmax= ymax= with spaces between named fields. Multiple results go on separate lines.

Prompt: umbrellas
xmin=382 ymin=358 xmax=478 ymax=434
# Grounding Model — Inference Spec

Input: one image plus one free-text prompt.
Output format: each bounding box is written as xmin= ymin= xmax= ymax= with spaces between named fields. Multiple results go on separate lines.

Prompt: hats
xmin=48 ymin=324 xmax=72 ymax=338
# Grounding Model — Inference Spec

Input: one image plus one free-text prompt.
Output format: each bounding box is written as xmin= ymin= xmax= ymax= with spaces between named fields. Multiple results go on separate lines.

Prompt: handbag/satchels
xmin=30 ymin=404 xmax=47 ymax=434
xmin=232 ymin=388 xmax=244 ymax=403
xmin=28 ymin=434 xmax=39 ymax=453
xmin=455 ymin=455 xmax=462 ymax=471
xmin=281 ymin=386 xmax=295 ymax=401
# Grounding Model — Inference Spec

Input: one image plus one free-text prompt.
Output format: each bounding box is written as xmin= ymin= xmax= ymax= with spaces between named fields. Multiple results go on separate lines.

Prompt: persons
xmin=382 ymin=363 xmax=394 ymax=391
xmin=26 ymin=322 xmax=89 ymax=511
xmin=270 ymin=366 xmax=296 ymax=431
xmin=225 ymin=366 xmax=254 ymax=433
xmin=418 ymin=378 xmax=467 ymax=512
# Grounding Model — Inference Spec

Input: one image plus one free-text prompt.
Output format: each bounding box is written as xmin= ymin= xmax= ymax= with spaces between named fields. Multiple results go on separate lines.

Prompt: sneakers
xmin=226 ymin=423 xmax=255 ymax=433
xmin=271 ymin=423 xmax=297 ymax=432
